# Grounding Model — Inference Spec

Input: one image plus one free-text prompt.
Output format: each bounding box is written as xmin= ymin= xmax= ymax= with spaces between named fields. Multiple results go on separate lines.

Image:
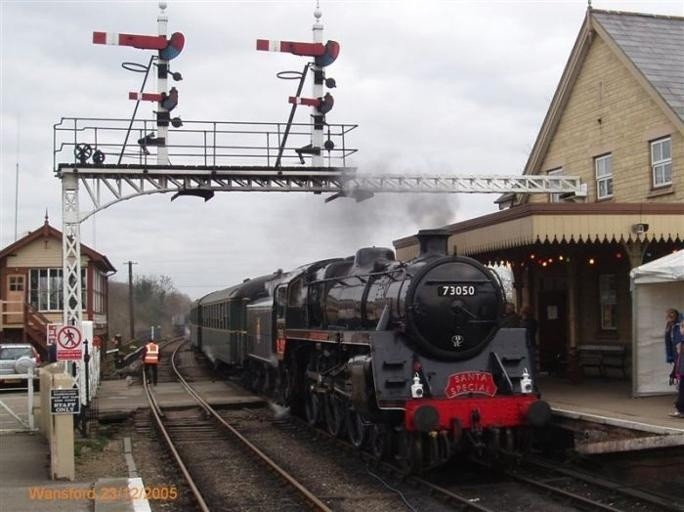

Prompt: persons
xmin=141 ymin=337 xmax=163 ymax=384
xmin=501 ymin=301 xmax=538 ymax=387
xmin=665 ymin=306 xmax=684 ymax=418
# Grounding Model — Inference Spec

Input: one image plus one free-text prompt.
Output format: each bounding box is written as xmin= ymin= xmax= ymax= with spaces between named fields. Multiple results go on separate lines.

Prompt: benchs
xmin=554 ymin=342 xmax=628 ymax=383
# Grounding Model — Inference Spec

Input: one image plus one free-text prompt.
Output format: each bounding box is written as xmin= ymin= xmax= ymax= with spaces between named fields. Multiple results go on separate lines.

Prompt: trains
xmin=189 ymin=229 xmax=552 ymax=478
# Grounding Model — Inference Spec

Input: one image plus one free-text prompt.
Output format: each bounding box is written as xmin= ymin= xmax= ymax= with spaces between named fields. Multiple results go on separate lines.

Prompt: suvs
xmin=0 ymin=343 xmax=43 ymax=391
